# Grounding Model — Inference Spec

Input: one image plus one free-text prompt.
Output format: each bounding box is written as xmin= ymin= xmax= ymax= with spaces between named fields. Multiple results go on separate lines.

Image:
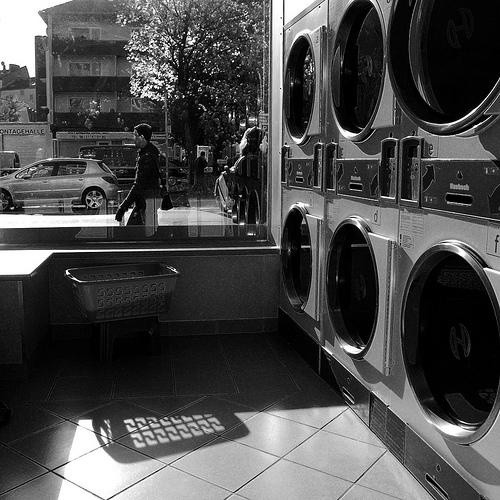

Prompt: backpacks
xmin=213 ymin=154 xmax=257 ymax=211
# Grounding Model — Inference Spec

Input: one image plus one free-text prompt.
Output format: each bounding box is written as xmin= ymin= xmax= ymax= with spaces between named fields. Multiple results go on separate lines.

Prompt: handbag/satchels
xmin=160 ymin=186 xmax=172 ymax=210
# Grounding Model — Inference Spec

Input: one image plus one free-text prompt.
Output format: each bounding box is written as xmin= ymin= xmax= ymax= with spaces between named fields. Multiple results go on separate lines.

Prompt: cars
xmin=0 ymin=157 xmax=120 ymax=212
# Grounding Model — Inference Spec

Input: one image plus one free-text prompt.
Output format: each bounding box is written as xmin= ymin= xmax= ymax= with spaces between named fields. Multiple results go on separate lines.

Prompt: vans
xmin=78 ymin=143 xmax=188 ymax=186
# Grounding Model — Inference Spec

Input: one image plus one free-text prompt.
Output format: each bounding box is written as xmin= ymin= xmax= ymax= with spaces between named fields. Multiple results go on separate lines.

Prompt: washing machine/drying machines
xmin=278 ymin=0 xmax=499 ymax=499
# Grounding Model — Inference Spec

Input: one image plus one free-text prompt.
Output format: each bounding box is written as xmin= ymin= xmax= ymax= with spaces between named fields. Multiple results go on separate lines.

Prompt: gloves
xmin=115 ymin=207 xmax=125 ymax=222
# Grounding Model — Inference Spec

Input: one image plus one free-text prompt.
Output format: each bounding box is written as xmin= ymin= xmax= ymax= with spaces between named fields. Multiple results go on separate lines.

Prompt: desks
xmin=1 ymin=249 xmax=53 ymax=401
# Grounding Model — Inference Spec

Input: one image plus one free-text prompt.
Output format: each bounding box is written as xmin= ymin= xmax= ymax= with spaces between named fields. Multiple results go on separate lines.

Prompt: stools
xmin=97 ymin=313 xmax=162 ymax=364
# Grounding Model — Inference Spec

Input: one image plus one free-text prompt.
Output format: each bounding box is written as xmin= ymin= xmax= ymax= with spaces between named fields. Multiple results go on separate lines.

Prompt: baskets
xmin=65 ymin=261 xmax=181 ymax=322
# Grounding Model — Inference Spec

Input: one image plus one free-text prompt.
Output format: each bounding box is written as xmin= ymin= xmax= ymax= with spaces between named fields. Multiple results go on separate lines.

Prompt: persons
xmin=232 ymin=127 xmax=268 ymax=225
xmin=186 ymin=149 xmax=213 ymax=199
xmin=113 ymin=123 xmax=167 ymax=226
